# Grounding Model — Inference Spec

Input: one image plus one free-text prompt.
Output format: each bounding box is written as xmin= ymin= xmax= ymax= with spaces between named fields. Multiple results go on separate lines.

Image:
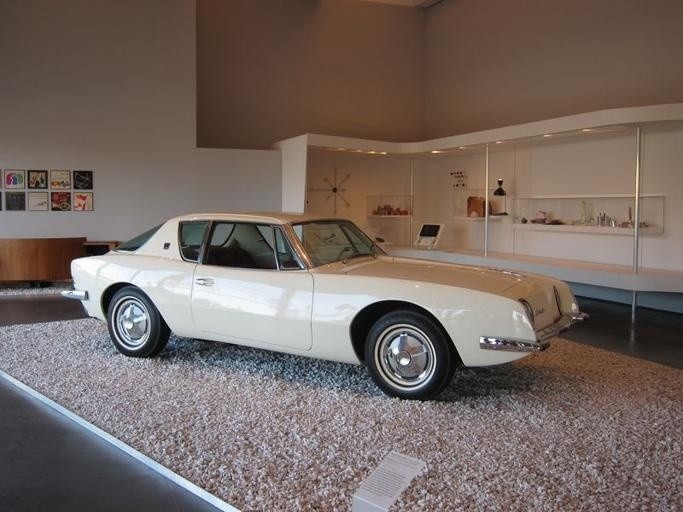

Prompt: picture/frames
xmin=1 ymin=169 xmax=93 ymax=212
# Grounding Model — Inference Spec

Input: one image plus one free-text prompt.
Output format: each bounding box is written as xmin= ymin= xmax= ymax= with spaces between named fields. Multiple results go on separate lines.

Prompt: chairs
xmin=181 ymin=245 xmax=256 ymax=268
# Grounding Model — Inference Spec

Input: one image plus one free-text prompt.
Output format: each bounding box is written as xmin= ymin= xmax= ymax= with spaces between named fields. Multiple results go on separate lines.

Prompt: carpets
xmin=1 ymin=319 xmax=682 ymax=512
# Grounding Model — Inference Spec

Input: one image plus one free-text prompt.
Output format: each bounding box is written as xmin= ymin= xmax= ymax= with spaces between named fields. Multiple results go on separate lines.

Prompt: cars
xmin=59 ymin=213 xmax=591 ymax=399
